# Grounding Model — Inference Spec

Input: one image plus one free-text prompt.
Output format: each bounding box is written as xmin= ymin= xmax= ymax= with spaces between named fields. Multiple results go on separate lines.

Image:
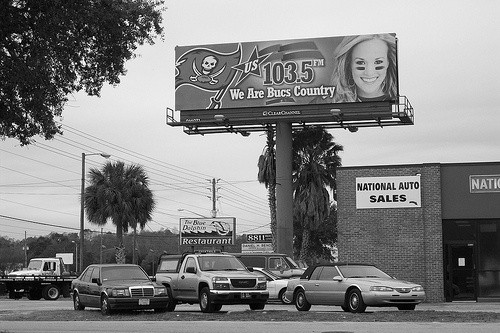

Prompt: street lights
xmin=71 ymin=241 xmax=77 ymax=274
xmin=79 ymin=151 xmax=111 ymax=275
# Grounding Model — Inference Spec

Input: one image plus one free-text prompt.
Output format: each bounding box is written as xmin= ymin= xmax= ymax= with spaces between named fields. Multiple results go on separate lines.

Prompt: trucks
xmin=7 ymin=256 xmax=77 ymax=300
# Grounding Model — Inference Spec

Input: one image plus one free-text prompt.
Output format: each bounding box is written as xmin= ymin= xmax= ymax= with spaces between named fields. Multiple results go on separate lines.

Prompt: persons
xmin=306 ymin=34 xmax=398 ymax=104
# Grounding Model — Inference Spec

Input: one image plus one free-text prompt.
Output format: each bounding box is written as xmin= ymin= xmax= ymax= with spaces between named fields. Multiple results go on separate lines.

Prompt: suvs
xmin=155 ymin=250 xmax=270 ymax=314
xmin=228 ymin=251 xmax=307 ymax=279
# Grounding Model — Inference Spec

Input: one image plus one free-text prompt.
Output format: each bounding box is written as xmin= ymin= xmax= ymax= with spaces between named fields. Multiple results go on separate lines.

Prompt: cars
xmin=70 ymin=263 xmax=169 ymax=317
xmin=245 ymin=266 xmax=293 ymax=305
xmin=286 ymin=262 xmax=426 ymax=314
